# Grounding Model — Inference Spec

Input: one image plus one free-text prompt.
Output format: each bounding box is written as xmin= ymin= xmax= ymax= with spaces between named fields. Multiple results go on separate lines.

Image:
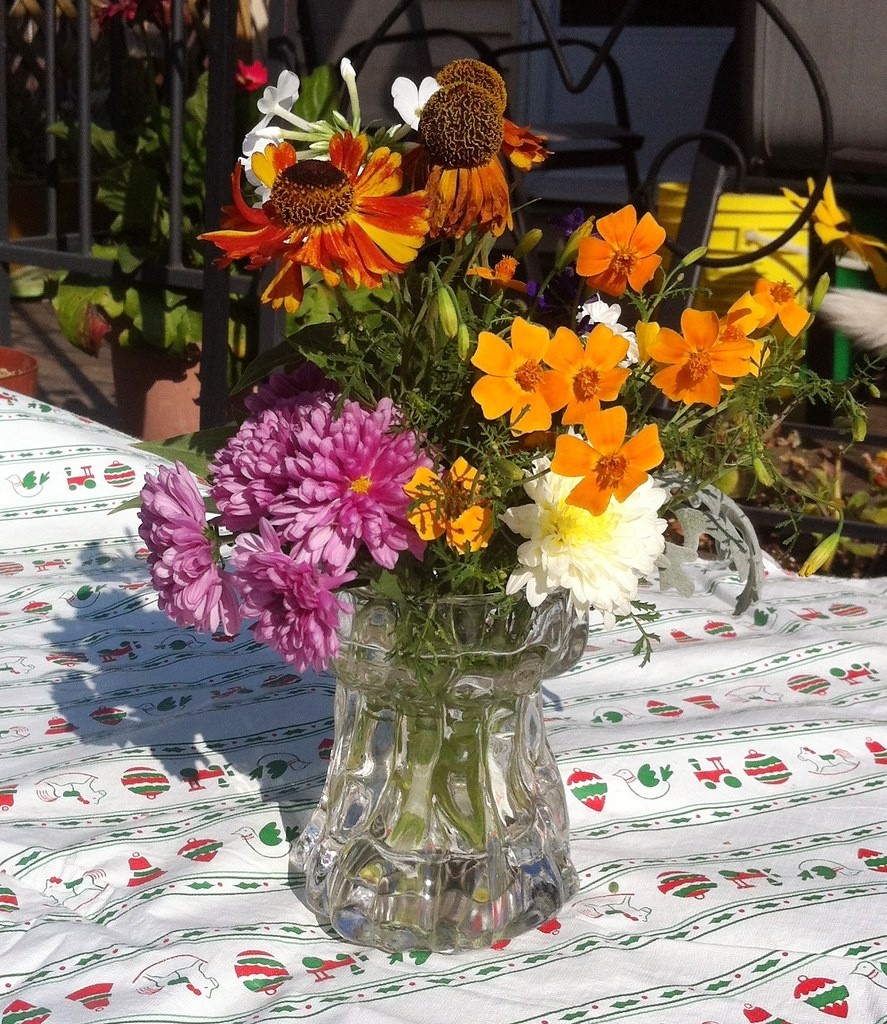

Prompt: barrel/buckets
xmin=657 ymin=182 xmax=870 ymax=399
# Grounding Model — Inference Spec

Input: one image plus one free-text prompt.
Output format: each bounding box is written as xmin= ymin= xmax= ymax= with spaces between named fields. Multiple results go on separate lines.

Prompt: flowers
xmin=108 ymin=58 xmax=886 ymax=859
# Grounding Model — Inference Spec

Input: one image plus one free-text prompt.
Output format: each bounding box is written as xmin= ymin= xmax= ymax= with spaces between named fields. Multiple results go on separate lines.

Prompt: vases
xmin=0 ymin=346 xmax=39 ymax=400
xmin=297 ymin=584 xmax=590 ymax=953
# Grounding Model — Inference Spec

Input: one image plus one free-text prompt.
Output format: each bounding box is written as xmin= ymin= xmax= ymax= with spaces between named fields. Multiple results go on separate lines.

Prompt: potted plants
xmin=8 ymin=64 xmax=338 ymax=442
